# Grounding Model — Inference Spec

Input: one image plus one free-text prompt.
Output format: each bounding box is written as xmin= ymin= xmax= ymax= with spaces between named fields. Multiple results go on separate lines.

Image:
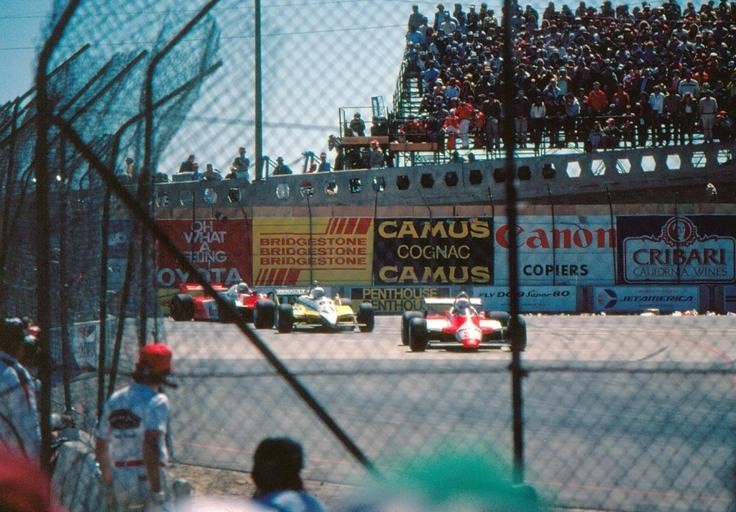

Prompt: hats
xmin=405 ymin=0 xmax=736 ymax=154
xmin=135 ymin=344 xmax=172 ymax=373
xmin=252 ymin=437 xmax=305 ymax=477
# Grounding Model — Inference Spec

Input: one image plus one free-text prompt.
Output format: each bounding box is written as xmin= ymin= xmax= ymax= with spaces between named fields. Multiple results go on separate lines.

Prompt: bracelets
xmin=151 ymin=489 xmax=164 ymax=502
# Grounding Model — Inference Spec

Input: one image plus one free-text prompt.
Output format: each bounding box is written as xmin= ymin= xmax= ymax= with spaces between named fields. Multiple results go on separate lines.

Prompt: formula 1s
xmin=400 ymin=292 xmax=527 ymax=351
xmin=171 ymin=278 xmax=374 ymax=333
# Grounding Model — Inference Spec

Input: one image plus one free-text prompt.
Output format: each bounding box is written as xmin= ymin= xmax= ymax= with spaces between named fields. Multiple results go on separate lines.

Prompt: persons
xmin=5 ymin=317 xmax=41 ymax=392
xmin=125 ymin=156 xmax=135 ymax=176
xmin=179 ymin=154 xmax=196 ymax=172
xmin=203 ymin=163 xmax=223 ymax=182
xmin=248 ymin=431 xmax=330 ymax=511
xmin=449 ymin=297 xmax=479 ymax=317
xmin=332 ymin=1 xmax=735 ymax=169
xmin=237 ymin=281 xmax=249 ymax=293
xmin=309 ymin=285 xmax=326 ymax=299
xmin=232 ymin=147 xmax=251 ymax=181
xmin=0 ymin=315 xmax=43 ymax=471
xmin=225 ymin=165 xmax=238 ymax=179
xmin=273 ymin=157 xmax=293 ymax=175
xmin=95 ymin=342 xmax=171 ymax=511
xmin=317 ymin=152 xmax=330 ymax=172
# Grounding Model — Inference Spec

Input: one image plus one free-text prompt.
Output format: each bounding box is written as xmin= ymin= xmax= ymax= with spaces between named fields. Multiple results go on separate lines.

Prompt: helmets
xmin=237 ymin=282 xmax=249 ymax=293
xmin=310 ymin=286 xmax=326 ymax=300
xmin=455 ymin=291 xmax=471 ymax=308
